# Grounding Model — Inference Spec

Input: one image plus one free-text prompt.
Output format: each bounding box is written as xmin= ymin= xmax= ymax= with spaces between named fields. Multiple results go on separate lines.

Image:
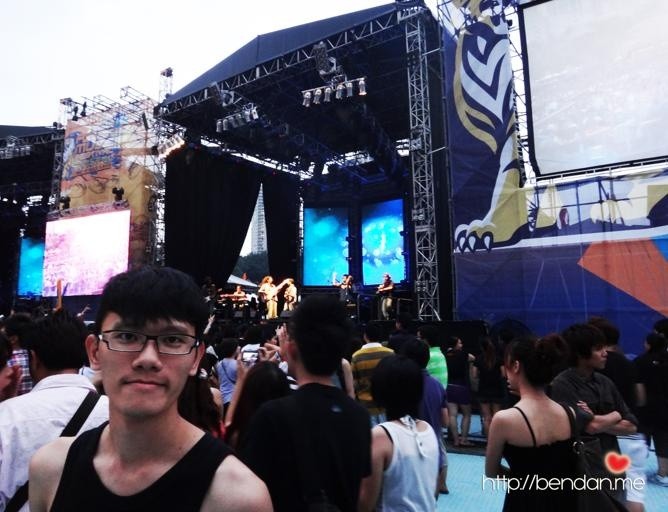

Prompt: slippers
xmin=454 ymin=443 xmax=474 ymax=448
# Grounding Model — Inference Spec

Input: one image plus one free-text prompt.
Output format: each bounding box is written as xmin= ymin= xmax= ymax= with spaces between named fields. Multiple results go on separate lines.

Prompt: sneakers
xmin=648 ymin=473 xmax=668 ymax=487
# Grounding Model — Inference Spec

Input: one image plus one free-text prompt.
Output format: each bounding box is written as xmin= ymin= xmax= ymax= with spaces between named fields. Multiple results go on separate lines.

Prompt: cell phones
xmin=242 ymin=351 xmax=260 ymax=362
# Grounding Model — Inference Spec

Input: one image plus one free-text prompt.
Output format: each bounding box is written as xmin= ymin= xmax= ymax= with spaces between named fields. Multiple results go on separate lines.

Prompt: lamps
xmin=300 ymin=58 xmax=367 ymax=107
xmin=216 ymin=96 xmax=258 ymax=134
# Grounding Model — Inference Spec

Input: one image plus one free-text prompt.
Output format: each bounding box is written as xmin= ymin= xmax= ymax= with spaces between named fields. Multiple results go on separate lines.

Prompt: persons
xmin=0 ymin=264 xmax=668 ymax=511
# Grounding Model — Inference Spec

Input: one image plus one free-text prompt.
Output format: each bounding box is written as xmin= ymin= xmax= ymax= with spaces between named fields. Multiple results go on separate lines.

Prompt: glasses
xmin=96 ymin=330 xmax=200 ymax=356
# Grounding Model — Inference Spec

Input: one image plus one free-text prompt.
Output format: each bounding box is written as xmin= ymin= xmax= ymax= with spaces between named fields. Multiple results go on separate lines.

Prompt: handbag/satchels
xmin=571 ymin=438 xmax=615 ymax=511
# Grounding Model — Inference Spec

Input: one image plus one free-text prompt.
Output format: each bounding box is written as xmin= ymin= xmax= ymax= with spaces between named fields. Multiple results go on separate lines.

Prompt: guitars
xmin=260 ymin=278 xmax=289 ymax=303
